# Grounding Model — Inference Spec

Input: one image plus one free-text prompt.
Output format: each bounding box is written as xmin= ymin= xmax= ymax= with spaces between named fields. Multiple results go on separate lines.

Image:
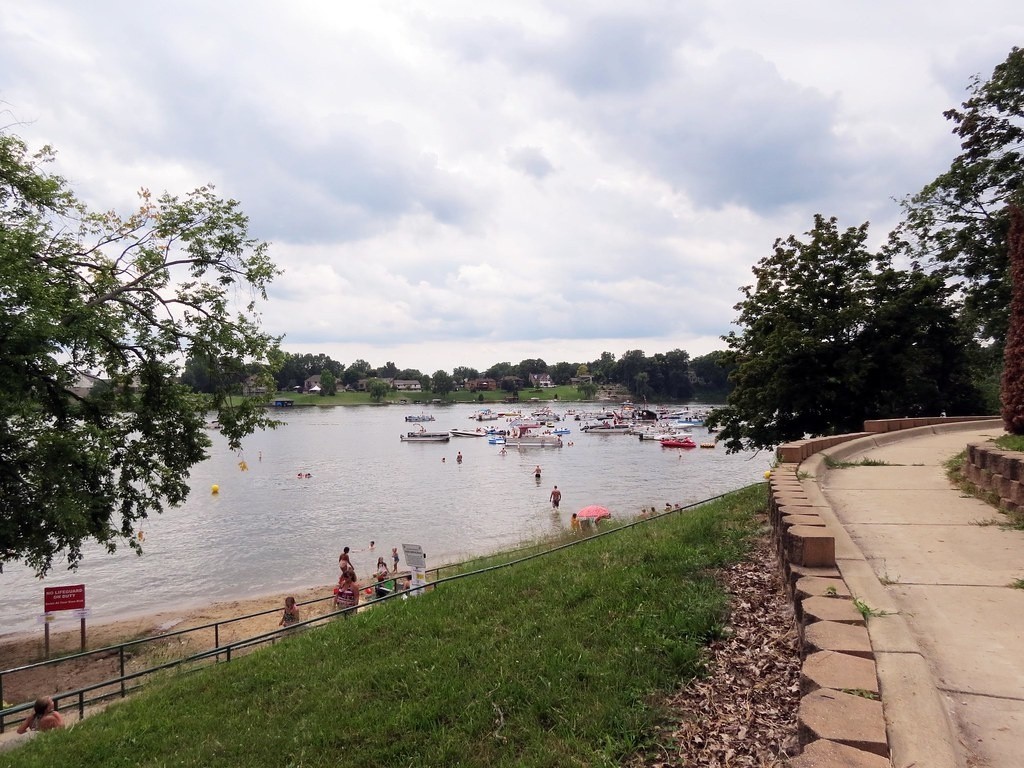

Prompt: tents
xmin=309 ymin=385 xmax=321 ymax=394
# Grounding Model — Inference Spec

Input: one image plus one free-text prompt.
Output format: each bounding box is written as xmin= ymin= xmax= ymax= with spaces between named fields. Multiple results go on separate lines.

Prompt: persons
xmin=665 ymin=503 xmax=678 ymax=511
xmin=279 ymin=597 xmax=299 ymax=627
xmin=571 ymin=514 xmax=579 ymax=528
xmin=339 ymin=547 xmax=354 ymax=573
xmin=375 ymin=576 xmax=384 ymax=596
xmin=550 ymin=485 xmax=562 ymax=508
xmin=369 ymin=541 xmax=375 ymax=549
xmin=499 ymin=448 xmax=507 ymax=454
xmin=392 ymin=548 xmax=399 ymax=574
xmin=373 ymin=557 xmax=390 ymax=578
xmin=333 ymin=571 xmax=359 ymax=616
xmin=456 ymin=452 xmax=462 ymax=461
xmin=531 ymin=466 xmax=542 ymax=478
xmin=17 ymin=697 xmax=63 ymax=734
xmin=595 ymin=513 xmax=610 ymax=525
xmin=404 ymin=575 xmax=412 ymax=591
xmin=419 ymin=405 xmax=709 ymax=445
xmin=639 ymin=507 xmax=660 ymax=519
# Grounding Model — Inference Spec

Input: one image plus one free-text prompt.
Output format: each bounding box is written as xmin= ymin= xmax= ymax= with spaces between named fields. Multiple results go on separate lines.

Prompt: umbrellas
xmin=577 ymin=505 xmax=608 ymax=517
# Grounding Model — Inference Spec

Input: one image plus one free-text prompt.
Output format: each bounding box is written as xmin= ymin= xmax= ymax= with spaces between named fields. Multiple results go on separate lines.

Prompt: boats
xmin=400 ymin=423 xmax=450 ymax=442
xmin=448 ymin=428 xmax=487 ymax=437
xmin=405 ymin=411 xmax=435 ymax=421
xmin=469 ymin=408 xmax=499 ymax=421
xmin=486 ymin=400 xmax=716 ymax=450
